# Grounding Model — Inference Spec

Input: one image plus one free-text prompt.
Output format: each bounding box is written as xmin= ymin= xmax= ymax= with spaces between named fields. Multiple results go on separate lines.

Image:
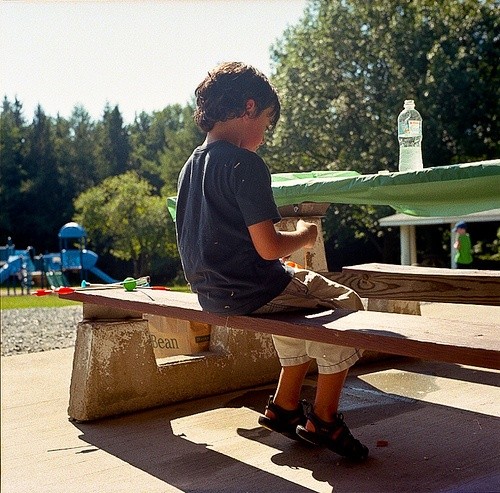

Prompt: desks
xmin=167 ymin=158 xmax=500 ymax=273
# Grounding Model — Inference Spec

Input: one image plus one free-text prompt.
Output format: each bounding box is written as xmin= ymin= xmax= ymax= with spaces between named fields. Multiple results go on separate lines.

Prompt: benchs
xmin=341 ymin=262 xmax=500 ymax=305
xmin=56 ymin=283 xmax=500 ymax=377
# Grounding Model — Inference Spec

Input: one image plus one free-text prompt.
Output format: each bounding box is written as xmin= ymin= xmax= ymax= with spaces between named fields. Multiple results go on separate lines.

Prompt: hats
xmin=452 ymin=222 xmax=466 ymax=232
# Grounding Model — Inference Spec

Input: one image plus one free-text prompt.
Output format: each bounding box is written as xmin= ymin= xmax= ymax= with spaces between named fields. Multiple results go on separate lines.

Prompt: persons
xmin=176 ymin=61 xmax=369 ymax=459
xmin=452 ymin=221 xmax=474 ymax=269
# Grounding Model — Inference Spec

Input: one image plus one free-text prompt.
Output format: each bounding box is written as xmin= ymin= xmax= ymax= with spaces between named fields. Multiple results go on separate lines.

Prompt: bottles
xmin=397 ymin=99 xmax=424 ymax=172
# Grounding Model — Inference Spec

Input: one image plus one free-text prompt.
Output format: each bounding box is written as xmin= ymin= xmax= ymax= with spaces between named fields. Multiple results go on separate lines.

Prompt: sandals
xmin=259 ymin=395 xmax=318 ymax=448
xmin=296 ymin=402 xmax=369 ymax=460
